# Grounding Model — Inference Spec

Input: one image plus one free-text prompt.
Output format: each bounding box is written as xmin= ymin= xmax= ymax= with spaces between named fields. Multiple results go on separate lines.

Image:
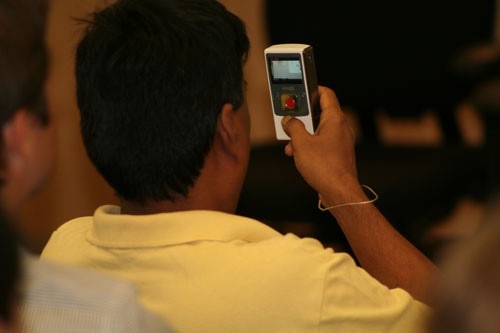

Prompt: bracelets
xmin=318 ymin=184 xmax=378 ymax=211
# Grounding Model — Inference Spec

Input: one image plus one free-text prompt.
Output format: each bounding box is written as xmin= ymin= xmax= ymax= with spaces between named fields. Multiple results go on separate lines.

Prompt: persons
xmin=0 ymin=0 xmax=178 ymax=333
xmin=426 ymin=0 xmax=500 ymax=332
xmin=40 ymin=0 xmax=442 ymax=333
